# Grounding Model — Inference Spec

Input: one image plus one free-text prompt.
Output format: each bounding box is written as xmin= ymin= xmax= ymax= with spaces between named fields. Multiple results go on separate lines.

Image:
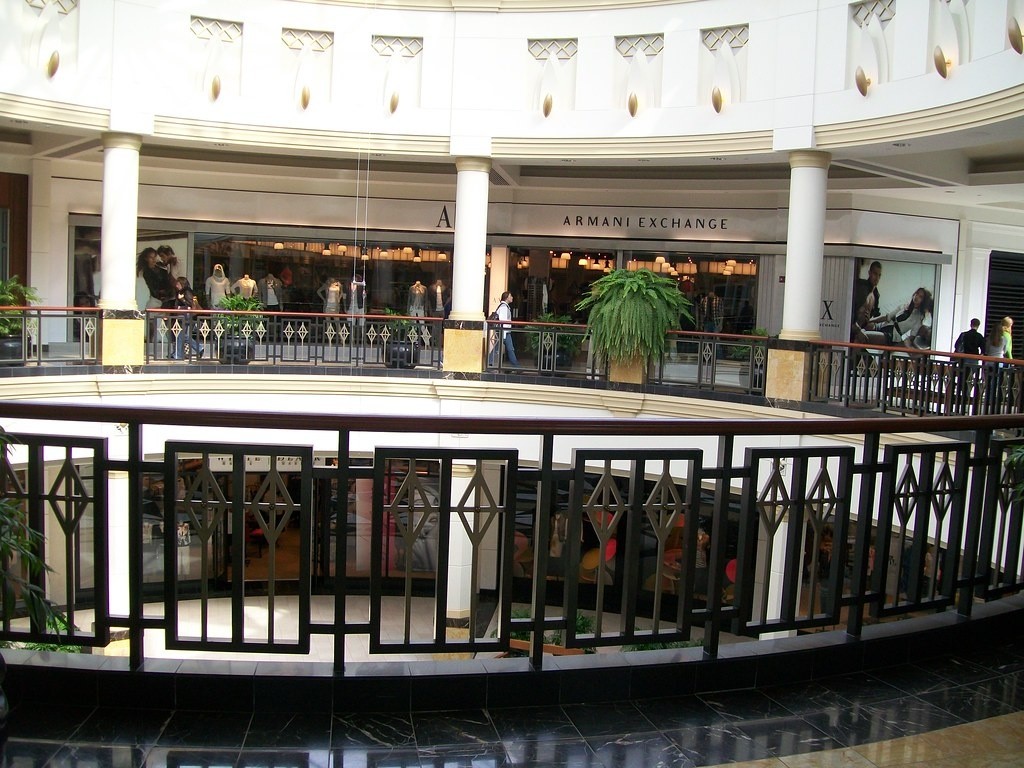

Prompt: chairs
xmin=246 ymin=520 xmax=281 ymax=558
xmin=662 ymin=513 xmax=684 ymax=596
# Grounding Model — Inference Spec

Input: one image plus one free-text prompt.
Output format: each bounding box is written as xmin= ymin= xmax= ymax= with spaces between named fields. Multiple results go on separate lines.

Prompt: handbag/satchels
xmin=487 ymin=312 xmax=499 ymax=325
xmin=951 ymin=332 xmax=964 ymax=363
xmin=191 ymin=296 xmax=203 ymax=317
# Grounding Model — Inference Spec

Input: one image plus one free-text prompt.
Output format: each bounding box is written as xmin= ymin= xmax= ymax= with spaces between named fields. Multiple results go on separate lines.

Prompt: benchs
xmin=882 ymin=359 xmax=975 ymax=405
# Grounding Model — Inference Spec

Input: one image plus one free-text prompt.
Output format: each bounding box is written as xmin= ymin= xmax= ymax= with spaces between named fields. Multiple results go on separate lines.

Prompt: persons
xmin=693 ymin=529 xmax=709 ymax=595
xmin=407 ymin=281 xmax=431 ymax=348
xmin=74 ymin=292 xmax=94 ymax=341
xmin=177 ymin=519 xmax=191 ymax=580
xmin=137 ymin=244 xmax=183 ymax=310
xmin=852 ymin=261 xmax=933 ymax=348
xmin=258 ymin=274 xmax=289 ymax=341
xmin=699 ymin=293 xmax=722 ymax=359
xmin=487 ymin=290 xmax=524 ymax=373
xmin=230 ymin=272 xmax=258 ymax=299
xmin=317 ymin=278 xmax=345 ymax=345
xmin=205 ymin=263 xmax=230 ymax=334
xmin=551 ymin=513 xmax=582 ymax=558
xmin=924 ymin=545 xmax=941 ymax=598
xmin=956 ymin=318 xmax=984 ymax=399
xmin=343 ymin=276 xmax=367 ymax=344
xmin=166 ymin=277 xmax=204 ymax=358
xmin=428 ymin=279 xmax=451 ymax=346
xmin=984 ymin=317 xmax=1014 ymax=398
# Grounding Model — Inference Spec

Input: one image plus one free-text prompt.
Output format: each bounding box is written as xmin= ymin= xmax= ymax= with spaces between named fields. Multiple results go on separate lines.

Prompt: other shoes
xmin=489 ymin=369 xmax=497 ymax=373
xmin=516 ymin=371 xmax=523 ymax=375
xmin=166 ymin=354 xmax=174 ymax=359
xmin=199 ymin=349 xmax=204 ymax=358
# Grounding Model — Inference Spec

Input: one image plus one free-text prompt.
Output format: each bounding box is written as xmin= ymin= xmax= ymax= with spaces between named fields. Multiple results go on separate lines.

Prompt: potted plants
xmin=0 ymin=274 xmax=44 ymax=367
xmin=573 ymin=268 xmax=696 ymax=383
xmin=729 ymin=327 xmax=780 ymax=396
xmin=381 ymin=307 xmax=419 ymax=370
xmin=525 ymin=313 xmax=582 ymax=376
xmin=212 ymin=296 xmax=264 ymax=365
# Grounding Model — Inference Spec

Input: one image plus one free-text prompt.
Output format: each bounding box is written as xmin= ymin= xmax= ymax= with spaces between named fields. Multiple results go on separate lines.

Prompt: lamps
xmin=518 ymin=256 xmax=527 ymax=269
xmin=551 ymin=251 xmax=571 ymax=269
xmin=578 ymin=255 xmax=587 ymax=266
xmin=337 ymin=244 xmax=348 ymax=252
xmin=273 ymin=241 xmax=284 ymax=249
xmin=592 ymin=258 xmax=600 ymax=268
xmin=403 ymin=246 xmax=412 ymax=254
xmin=656 ymin=256 xmax=737 ymax=283
xmin=413 ymin=245 xmax=421 ymax=263
xmin=322 ymin=243 xmax=331 ymax=256
xmin=603 ymin=260 xmax=610 ymax=272
xmin=438 ymin=251 xmax=446 ymax=260
xmin=379 ymin=249 xmax=388 ymax=257
xmin=361 ymin=248 xmax=369 ymax=260
